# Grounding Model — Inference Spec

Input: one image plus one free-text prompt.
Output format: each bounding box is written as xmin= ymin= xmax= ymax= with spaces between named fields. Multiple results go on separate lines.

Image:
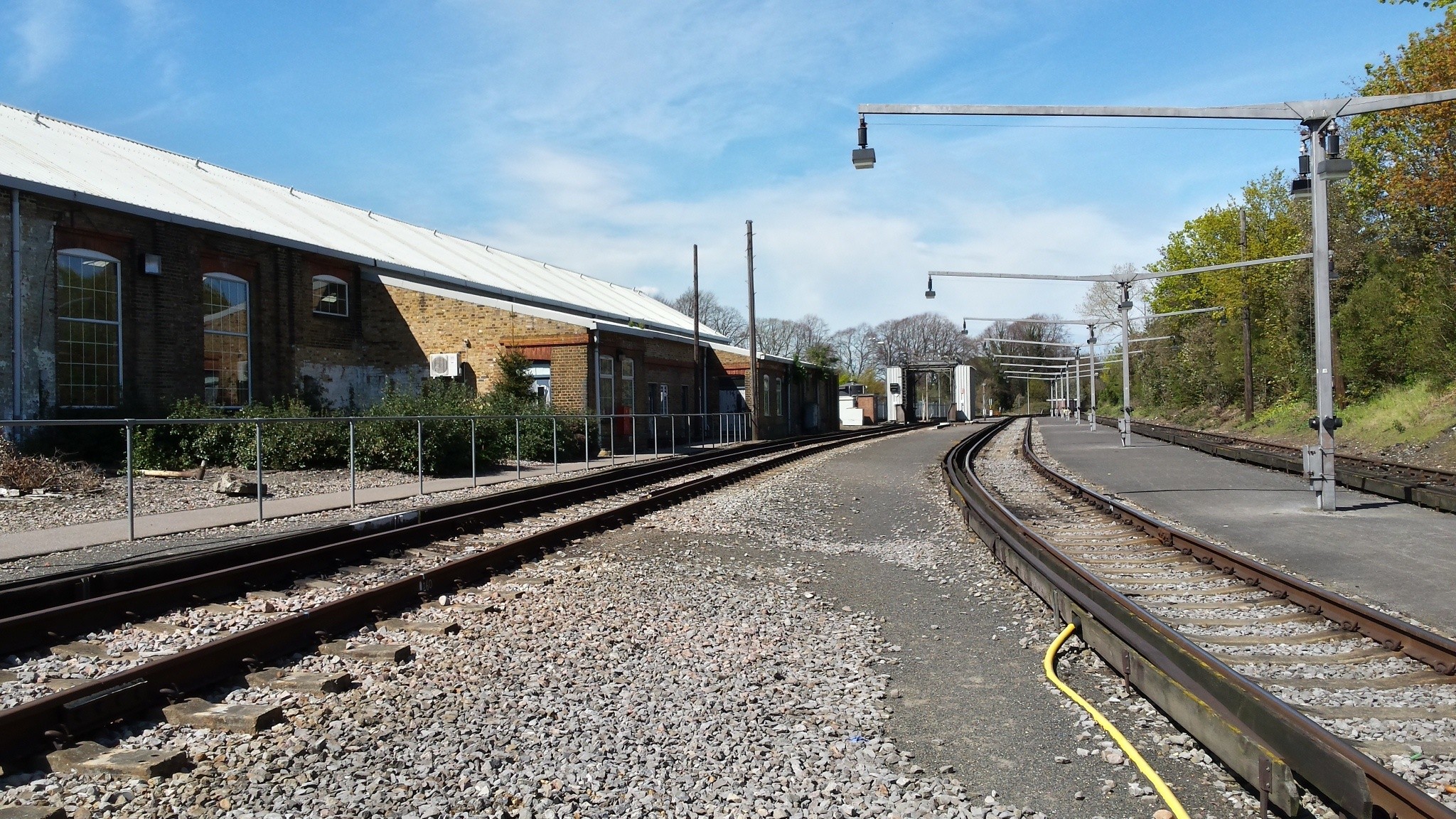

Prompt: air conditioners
xmin=429 ymin=352 xmax=462 ymax=380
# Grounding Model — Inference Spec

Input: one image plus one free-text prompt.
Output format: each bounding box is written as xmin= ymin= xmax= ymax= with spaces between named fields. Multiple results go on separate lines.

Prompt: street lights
xmin=850 ymin=88 xmax=1456 ymax=513
xmin=1027 ymin=369 xmax=1034 ymax=416
xmin=958 ymin=305 xmax=1229 ymax=430
xmin=980 ymin=335 xmax=1179 ymax=425
xmin=868 ymin=337 xmax=884 ymax=394
xmin=925 ymin=248 xmax=1341 ymax=449
